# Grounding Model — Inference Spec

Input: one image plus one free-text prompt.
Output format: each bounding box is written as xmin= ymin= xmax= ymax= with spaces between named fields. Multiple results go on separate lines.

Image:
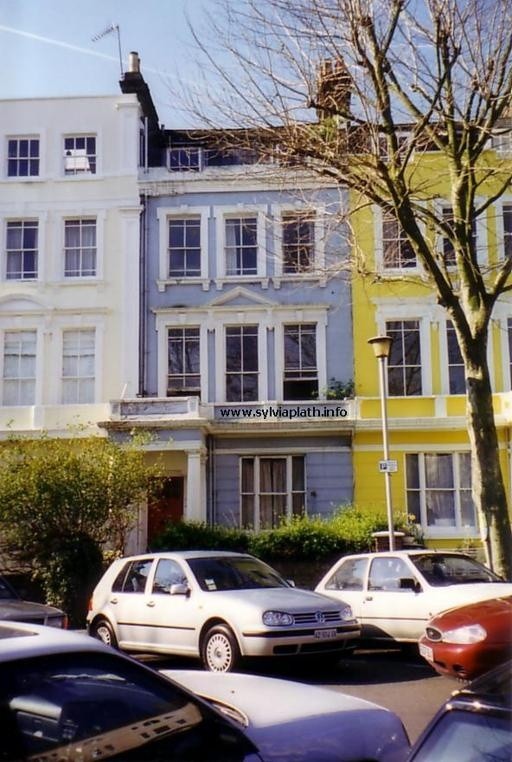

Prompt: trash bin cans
xmin=370 ymin=531 xmax=405 ymax=552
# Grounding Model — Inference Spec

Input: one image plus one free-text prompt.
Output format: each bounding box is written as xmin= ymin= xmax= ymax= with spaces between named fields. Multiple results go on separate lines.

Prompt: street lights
xmin=367 ymin=331 xmax=400 ymax=553
